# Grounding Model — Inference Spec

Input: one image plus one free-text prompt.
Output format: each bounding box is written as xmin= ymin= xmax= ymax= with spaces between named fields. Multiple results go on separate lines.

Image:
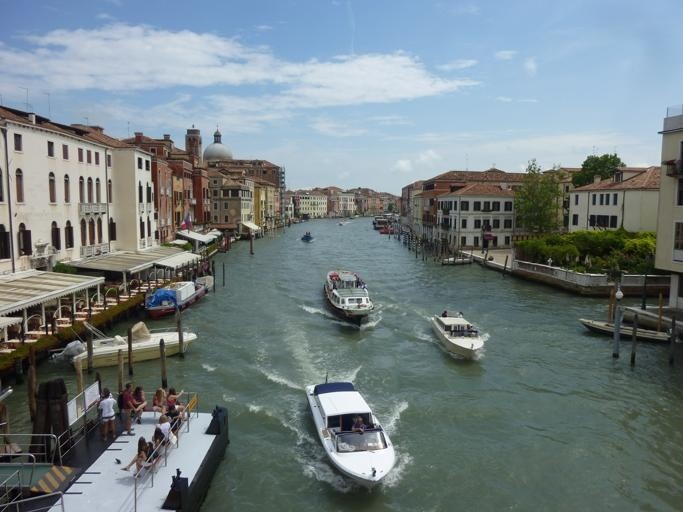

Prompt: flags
xmin=188 ymin=211 xmax=193 ymax=230
xmin=181 ymin=209 xmax=186 ymax=230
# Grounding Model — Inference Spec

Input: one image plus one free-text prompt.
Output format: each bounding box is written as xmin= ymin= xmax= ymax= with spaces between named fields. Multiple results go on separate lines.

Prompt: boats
xmin=301 ymin=231 xmax=313 ymax=242
xmin=430 ymin=314 xmax=483 ymax=359
xmin=372 ymin=214 xmax=399 ymax=234
xmin=144 ymin=276 xmax=214 ymax=318
xmin=578 ymin=317 xmax=671 ymax=343
xmin=323 ymin=270 xmax=374 ymax=321
xmin=51 ymin=321 xmax=197 ymax=371
xmin=304 ymin=381 xmax=395 ymax=491
xmin=442 ymin=257 xmax=469 ymax=265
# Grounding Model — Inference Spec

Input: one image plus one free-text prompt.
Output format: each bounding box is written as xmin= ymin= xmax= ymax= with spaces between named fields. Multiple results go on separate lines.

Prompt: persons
xmin=458 ymin=312 xmax=463 ymax=317
xmin=98 ymin=382 xmax=186 ymax=477
xmin=330 ymin=271 xmax=339 ymax=289
xmin=352 ymin=272 xmax=368 ymax=288
xmin=442 ymin=310 xmax=448 ymax=316
xmin=352 ymin=417 xmax=367 ymax=433
